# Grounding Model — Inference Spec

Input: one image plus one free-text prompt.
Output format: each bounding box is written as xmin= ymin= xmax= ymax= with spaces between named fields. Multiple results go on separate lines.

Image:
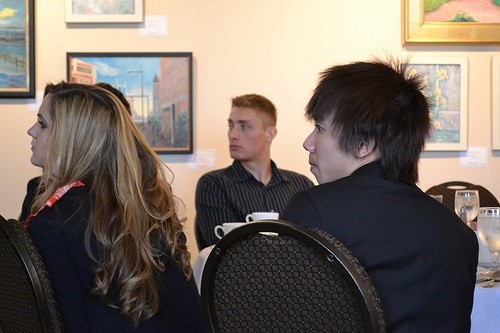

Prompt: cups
xmin=214 ymin=222 xmax=246 ymax=240
xmin=245 ymin=212 xmax=279 ymax=236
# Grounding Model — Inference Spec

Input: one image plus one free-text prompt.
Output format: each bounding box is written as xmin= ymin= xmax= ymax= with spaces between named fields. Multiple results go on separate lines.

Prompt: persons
xmin=19 ymin=83 xmax=131 ymax=221
xmin=195 ymin=94 xmax=315 ymax=251
xmin=25 ymin=80 xmax=204 ymax=333
xmin=278 ymin=61 xmax=479 ymax=333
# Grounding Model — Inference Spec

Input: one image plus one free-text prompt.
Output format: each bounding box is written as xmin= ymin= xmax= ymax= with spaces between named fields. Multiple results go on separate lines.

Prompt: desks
xmin=194 ymin=227 xmax=500 ymax=333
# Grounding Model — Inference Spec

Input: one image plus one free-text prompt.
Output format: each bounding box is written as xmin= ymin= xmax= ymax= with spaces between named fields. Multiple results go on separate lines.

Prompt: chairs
xmin=424 ymin=181 xmax=499 ymax=223
xmin=198 ymin=219 xmax=386 ymax=332
xmin=0 ymin=216 xmax=67 ymax=333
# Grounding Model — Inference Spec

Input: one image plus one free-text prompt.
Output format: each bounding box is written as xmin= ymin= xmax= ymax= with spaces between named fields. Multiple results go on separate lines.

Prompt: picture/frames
xmin=65 ymin=51 xmax=195 ymax=155
xmin=65 ymin=0 xmax=144 ymax=24
xmin=0 ymin=0 xmax=38 ymax=99
xmin=387 ymin=57 xmax=469 ymax=153
xmin=401 ymin=0 xmax=500 ymax=46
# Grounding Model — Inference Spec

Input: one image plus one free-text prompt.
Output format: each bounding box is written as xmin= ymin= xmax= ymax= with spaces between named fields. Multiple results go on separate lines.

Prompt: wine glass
xmin=454 ymin=190 xmax=480 ymax=229
xmin=478 ymin=207 xmax=500 ymax=279
